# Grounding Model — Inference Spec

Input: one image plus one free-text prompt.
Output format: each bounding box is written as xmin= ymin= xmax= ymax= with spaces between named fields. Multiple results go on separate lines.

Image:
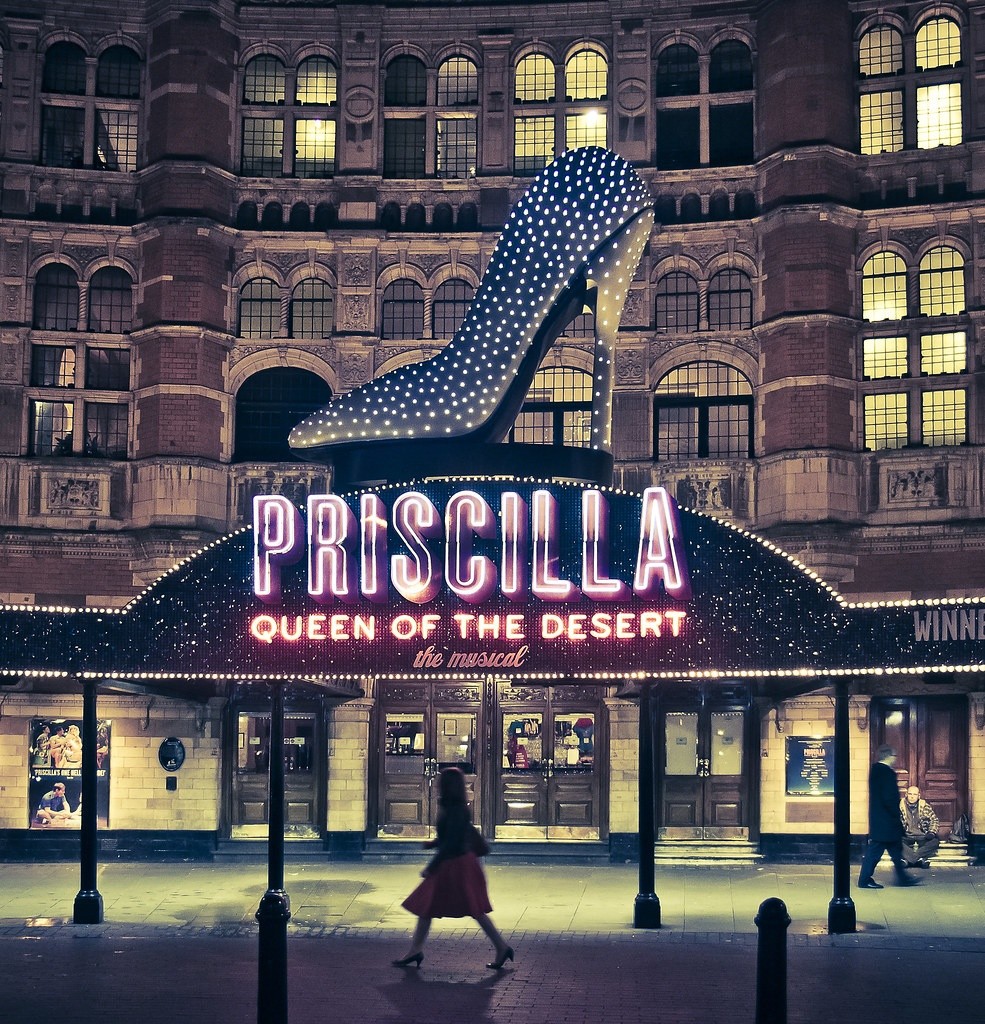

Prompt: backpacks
xmin=948 ymin=814 xmax=969 ymax=844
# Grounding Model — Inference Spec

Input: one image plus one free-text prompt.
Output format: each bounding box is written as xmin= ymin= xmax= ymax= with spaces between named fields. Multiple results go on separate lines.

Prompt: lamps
xmin=858 ymin=707 xmax=867 ymax=729
xmin=974 ymin=702 xmax=985 ymax=730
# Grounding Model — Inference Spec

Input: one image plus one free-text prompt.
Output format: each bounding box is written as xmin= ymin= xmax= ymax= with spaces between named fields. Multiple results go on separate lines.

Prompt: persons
xmin=32 ymin=720 xmax=109 ymax=768
xmin=390 ymin=767 xmax=514 ymax=968
xmin=857 ymin=747 xmax=939 ymax=889
xmin=37 ymin=782 xmax=82 ymax=823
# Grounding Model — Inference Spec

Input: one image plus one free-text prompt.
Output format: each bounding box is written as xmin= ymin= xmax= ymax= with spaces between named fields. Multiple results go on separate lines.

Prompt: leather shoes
xmin=858 ymin=878 xmax=884 ymax=888
xmin=894 ymin=875 xmax=924 ymax=887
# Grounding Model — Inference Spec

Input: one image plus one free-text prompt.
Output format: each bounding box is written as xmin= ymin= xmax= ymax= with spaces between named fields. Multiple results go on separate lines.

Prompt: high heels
xmin=286 ymin=148 xmax=656 ymax=451
xmin=391 ymin=953 xmax=425 ymax=968
xmin=486 ymin=948 xmax=514 ymax=968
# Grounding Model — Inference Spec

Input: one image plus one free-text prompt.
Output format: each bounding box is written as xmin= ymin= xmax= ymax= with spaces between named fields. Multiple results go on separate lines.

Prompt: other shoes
xmin=918 ymin=857 xmax=930 ymax=869
xmin=900 ymin=859 xmax=909 ymax=868
